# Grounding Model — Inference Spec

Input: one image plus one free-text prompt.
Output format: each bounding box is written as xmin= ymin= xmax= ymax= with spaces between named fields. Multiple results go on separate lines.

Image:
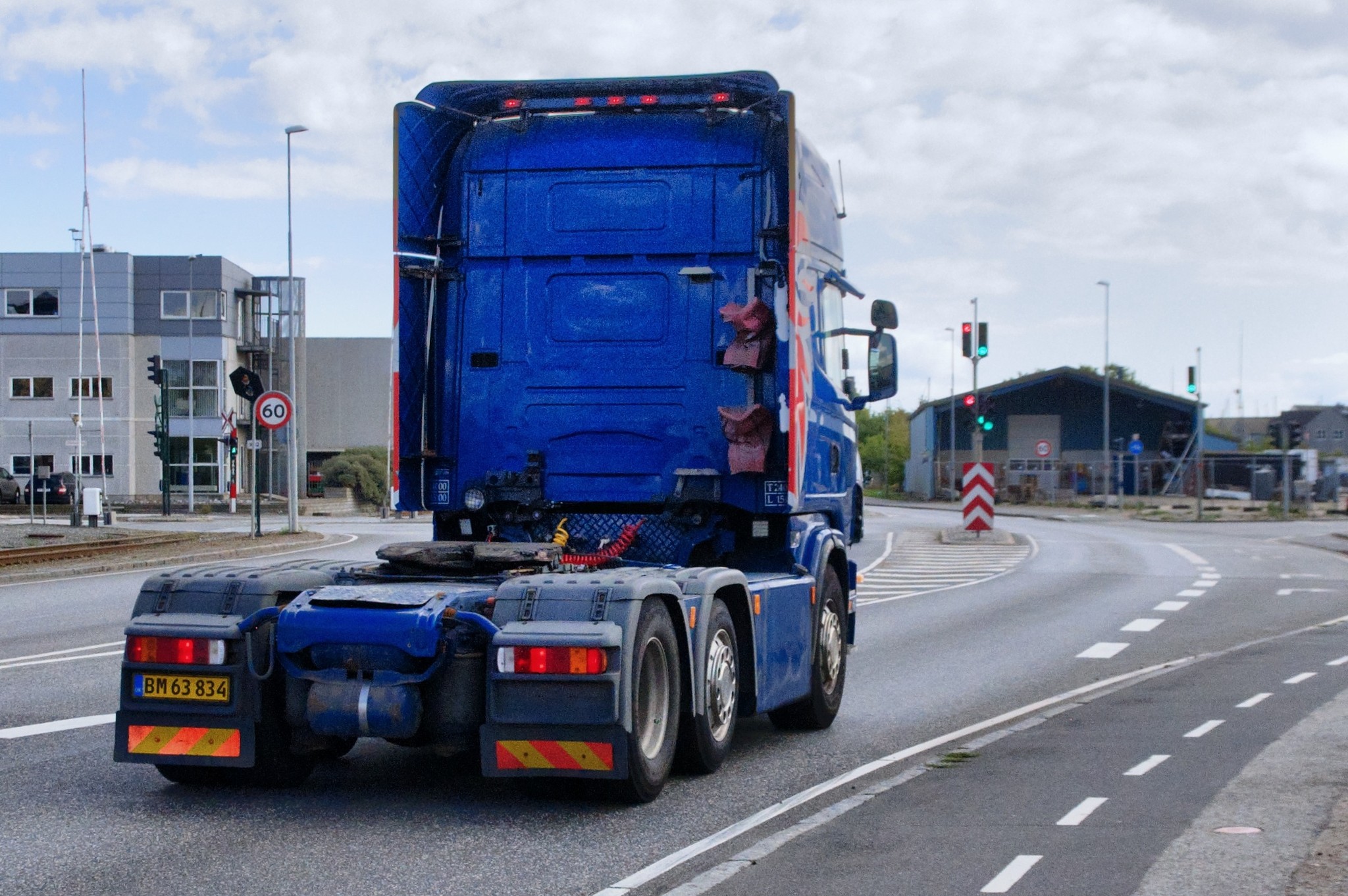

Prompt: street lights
xmin=287 ymin=123 xmax=311 ymax=535
xmin=1096 ymin=281 xmax=1110 ymax=506
xmin=945 ymin=326 xmax=956 ymax=499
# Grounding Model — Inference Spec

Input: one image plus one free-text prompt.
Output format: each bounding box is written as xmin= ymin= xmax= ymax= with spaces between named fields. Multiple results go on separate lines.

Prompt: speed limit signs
xmin=252 ymin=391 xmax=291 ymax=429
xmin=1036 ymin=440 xmax=1052 ymax=457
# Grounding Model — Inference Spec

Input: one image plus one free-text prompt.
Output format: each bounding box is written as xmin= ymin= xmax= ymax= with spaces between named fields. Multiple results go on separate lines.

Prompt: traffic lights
xmin=1187 ymin=364 xmax=1197 ymax=393
xmin=229 ymin=366 xmax=264 ymax=404
xmin=145 ymin=355 xmax=163 ymax=386
xmin=1268 ymin=422 xmax=1283 ymax=448
xmin=229 ymin=436 xmax=239 ymax=455
xmin=1288 ymin=423 xmax=1303 ymax=450
xmin=963 ymin=394 xmax=976 ymax=405
xmin=962 ymin=322 xmax=973 ymax=358
xmin=147 ymin=431 xmax=163 ymax=457
xmin=218 ymin=438 xmax=228 ymax=445
xmin=976 ymin=394 xmax=994 ymax=435
xmin=978 ymin=323 xmax=989 ymax=358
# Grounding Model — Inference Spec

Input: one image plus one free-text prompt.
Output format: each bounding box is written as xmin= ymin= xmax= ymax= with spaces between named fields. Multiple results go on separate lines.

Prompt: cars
xmin=25 ymin=471 xmax=85 ymax=505
xmin=0 ymin=467 xmax=21 ymax=504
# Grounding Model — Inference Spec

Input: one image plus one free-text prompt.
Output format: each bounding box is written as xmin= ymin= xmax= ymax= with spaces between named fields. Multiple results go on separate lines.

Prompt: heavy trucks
xmin=118 ymin=70 xmax=898 ymax=805
